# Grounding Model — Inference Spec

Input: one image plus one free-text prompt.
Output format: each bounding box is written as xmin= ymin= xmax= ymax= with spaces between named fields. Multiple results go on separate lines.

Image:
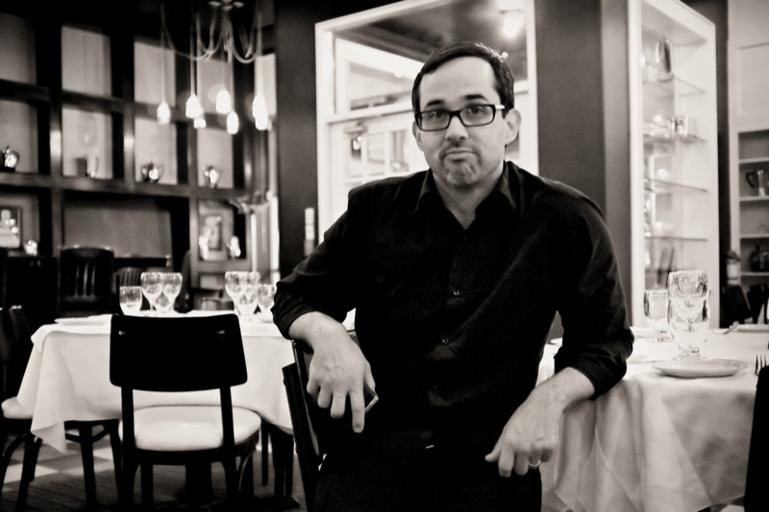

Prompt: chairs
xmin=743 ymin=366 xmax=769 ymax=512
xmin=0 ymin=245 xmax=362 ymax=512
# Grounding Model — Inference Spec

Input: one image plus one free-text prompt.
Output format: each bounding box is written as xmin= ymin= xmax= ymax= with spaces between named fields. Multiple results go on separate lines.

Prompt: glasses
xmin=415 ymin=98 xmax=505 ymax=133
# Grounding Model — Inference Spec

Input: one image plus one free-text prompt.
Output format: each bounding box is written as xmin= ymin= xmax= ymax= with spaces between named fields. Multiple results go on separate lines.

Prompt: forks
xmin=753 ymin=353 xmax=767 ymax=376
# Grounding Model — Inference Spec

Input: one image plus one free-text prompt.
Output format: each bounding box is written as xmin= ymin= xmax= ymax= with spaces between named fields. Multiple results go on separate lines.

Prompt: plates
xmin=653 ymin=357 xmax=744 ymax=378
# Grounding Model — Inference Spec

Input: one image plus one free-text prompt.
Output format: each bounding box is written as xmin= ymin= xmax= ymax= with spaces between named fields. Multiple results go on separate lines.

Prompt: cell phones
xmin=316 ymin=380 xmax=380 ymax=415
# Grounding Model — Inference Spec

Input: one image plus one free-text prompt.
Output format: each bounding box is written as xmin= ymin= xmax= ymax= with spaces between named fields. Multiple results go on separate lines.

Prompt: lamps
xmin=155 ymin=1 xmax=271 ymax=137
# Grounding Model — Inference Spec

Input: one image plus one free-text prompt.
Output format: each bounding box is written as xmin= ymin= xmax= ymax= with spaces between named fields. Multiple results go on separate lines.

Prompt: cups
xmin=119 ymin=285 xmax=142 ymax=316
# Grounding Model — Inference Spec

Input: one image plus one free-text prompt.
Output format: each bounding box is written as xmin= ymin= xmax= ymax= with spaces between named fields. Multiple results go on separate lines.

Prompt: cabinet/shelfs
xmin=600 ymin=1 xmax=769 ymax=329
xmin=0 ymin=1 xmax=257 ymax=310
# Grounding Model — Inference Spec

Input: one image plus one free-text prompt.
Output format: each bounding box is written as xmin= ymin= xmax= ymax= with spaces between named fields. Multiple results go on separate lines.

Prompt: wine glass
xmin=143 ymin=271 xmax=183 ymax=318
xmin=224 ymin=271 xmax=277 ymax=326
xmin=643 ymin=271 xmax=709 ymax=356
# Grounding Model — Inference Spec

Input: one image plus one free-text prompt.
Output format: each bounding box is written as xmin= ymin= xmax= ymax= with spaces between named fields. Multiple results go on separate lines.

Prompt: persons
xmin=270 ymin=37 xmax=636 ymax=509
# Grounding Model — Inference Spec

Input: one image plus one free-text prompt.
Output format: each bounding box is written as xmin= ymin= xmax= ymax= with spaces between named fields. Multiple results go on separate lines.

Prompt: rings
xmin=527 ymin=458 xmax=542 ymax=468
